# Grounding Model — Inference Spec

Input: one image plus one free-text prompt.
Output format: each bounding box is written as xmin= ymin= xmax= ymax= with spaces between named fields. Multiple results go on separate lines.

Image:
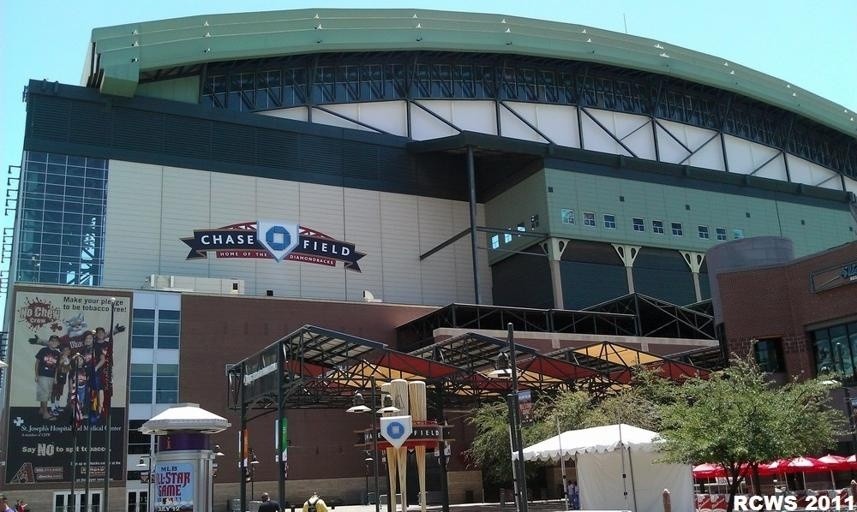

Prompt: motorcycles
xmin=13 ymin=498 xmax=32 ymax=512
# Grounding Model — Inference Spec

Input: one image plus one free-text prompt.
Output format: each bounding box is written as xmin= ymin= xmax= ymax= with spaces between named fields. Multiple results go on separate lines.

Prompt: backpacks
xmin=308 ymin=498 xmax=320 ymax=512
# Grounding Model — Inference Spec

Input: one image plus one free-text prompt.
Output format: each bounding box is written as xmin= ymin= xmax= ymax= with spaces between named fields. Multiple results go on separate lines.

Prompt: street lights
xmin=343 ymin=374 xmax=401 ymax=512
xmin=496 ymin=320 xmax=532 ymax=512
xmin=245 ymin=447 xmax=260 ymax=501
xmin=818 ymin=342 xmax=857 ymax=444
xmin=360 ymin=450 xmax=376 ymax=507
xmin=133 ymin=456 xmax=154 ymax=512
xmin=201 ymin=445 xmax=225 ymax=512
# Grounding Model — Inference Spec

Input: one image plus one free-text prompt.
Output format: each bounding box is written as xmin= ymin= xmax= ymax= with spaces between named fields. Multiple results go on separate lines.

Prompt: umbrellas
xmin=693 ymin=451 xmax=856 ymax=488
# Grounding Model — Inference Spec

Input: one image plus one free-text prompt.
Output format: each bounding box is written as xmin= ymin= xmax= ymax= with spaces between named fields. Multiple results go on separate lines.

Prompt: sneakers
xmin=38 ymin=406 xmax=64 ymax=422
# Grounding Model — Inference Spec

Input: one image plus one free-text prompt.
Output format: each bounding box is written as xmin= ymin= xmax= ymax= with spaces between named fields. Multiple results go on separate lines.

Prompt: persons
xmin=27 ymin=315 xmax=126 ymax=430
xmin=0 ymin=493 xmax=32 ymax=512
xmin=257 ymin=492 xmax=282 ymax=512
xmin=572 ymin=480 xmax=578 ymax=509
xmin=301 ymin=491 xmax=328 ymax=511
xmin=567 ymin=480 xmax=574 ymax=507
